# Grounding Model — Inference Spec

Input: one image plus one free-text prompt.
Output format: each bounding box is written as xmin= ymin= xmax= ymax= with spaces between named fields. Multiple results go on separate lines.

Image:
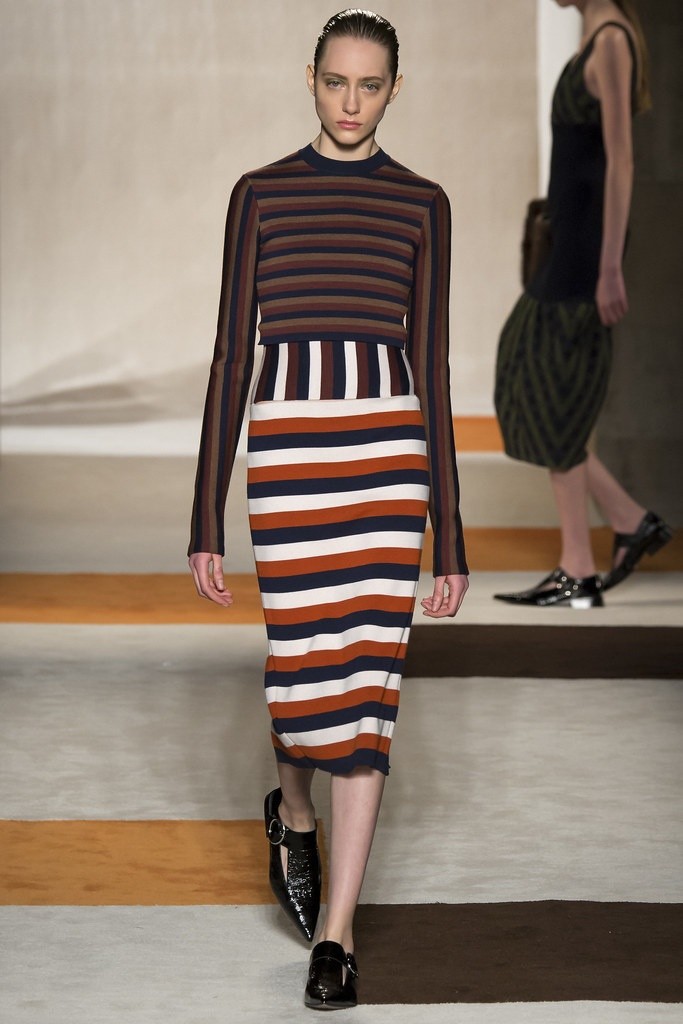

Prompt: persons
xmin=493 ymin=0 xmax=672 ymax=609
xmin=187 ymin=9 xmax=470 ymax=1010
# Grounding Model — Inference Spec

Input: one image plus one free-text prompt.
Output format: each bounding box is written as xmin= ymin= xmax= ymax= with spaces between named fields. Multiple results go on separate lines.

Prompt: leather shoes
xmin=262 ymin=787 xmax=322 ymax=942
xmin=304 ymin=941 xmax=359 ymax=1010
xmin=602 ymin=512 xmax=675 ymax=589
xmin=492 ymin=566 xmax=606 ymax=607
